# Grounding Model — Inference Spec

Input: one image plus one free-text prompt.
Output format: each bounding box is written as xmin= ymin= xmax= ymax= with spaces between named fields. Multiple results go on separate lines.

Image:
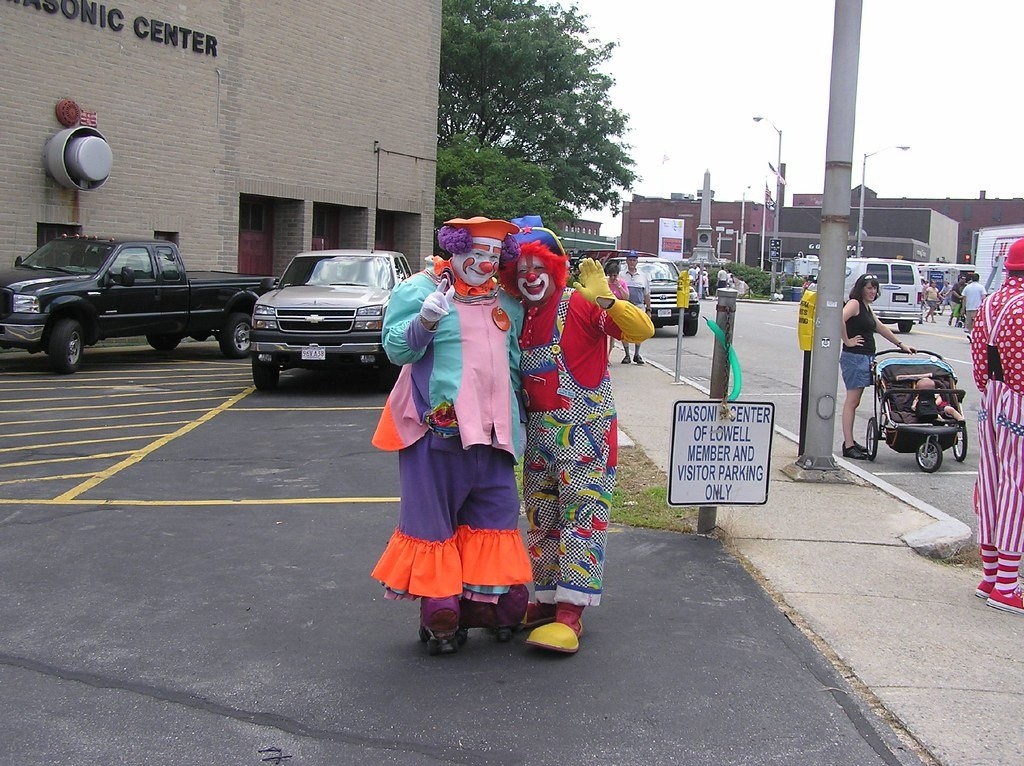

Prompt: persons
xmin=781 ymin=272 xmax=818 ymax=306
xmin=606 ymin=250 xmax=652 ymax=366
xmin=715 ymin=266 xmax=745 ymax=300
xmin=842 ymin=273 xmax=917 ymax=460
xmin=371 ymin=217 xmax=533 ymax=656
xmin=913 ymin=271 xmax=967 ymax=326
xmin=688 ymin=264 xmax=709 ymax=297
xmin=961 ymin=273 xmax=988 ymax=343
xmin=495 ymin=216 xmax=656 ymax=652
xmin=971 ymin=237 xmax=1024 ymax=615
xmin=911 ymin=378 xmax=964 ymax=421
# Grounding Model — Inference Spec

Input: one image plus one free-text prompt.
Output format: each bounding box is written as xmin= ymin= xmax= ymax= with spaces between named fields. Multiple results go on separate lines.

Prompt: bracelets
xmin=646 ymin=307 xmax=651 ymax=312
xmin=897 ymin=342 xmax=902 ymax=347
xmin=617 ymin=285 xmax=621 ymax=289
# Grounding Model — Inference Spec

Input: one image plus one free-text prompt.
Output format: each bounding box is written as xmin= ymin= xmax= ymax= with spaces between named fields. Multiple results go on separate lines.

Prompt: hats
xmin=1004 ymin=238 xmax=1024 ymax=270
xmin=626 ymin=249 xmax=638 ymax=257
xmin=443 ymin=217 xmax=520 ymax=241
xmin=511 ymin=216 xmax=565 ymax=256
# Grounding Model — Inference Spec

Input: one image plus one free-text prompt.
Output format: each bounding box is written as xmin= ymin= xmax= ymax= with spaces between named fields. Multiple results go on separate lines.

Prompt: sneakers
xmin=622 ymin=355 xmax=644 ymax=364
xmin=842 ymin=440 xmax=868 ymax=459
xmin=975 ymin=580 xmax=993 ymax=599
xmin=985 ymin=587 xmax=1024 ymax=615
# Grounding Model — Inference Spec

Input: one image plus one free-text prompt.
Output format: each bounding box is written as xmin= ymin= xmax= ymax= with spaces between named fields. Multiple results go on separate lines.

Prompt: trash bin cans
xmin=792 ymin=286 xmax=802 ymax=302
xmin=783 ymin=286 xmax=792 ymax=301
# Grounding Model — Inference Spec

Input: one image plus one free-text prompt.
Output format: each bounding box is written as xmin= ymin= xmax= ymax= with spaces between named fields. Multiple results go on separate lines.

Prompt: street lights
xmin=740 ymin=186 xmax=751 ymax=263
xmin=855 ymin=146 xmax=909 ymax=255
xmin=754 ymin=117 xmax=783 ymax=301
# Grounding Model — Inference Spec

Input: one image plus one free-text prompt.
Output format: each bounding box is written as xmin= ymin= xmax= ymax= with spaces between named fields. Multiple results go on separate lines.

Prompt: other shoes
xmin=948 ymin=321 xmax=952 ymax=326
xmin=967 ymin=334 xmax=972 ymax=343
xmin=525 ymin=616 xmax=583 ymax=653
xmin=955 ymin=324 xmax=959 ymax=327
xmin=938 ymin=312 xmax=942 ymax=316
xmin=931 ymin=321 xmax=936 ymax=323
xmin=522 ymin=602 xmax=556 ymax=628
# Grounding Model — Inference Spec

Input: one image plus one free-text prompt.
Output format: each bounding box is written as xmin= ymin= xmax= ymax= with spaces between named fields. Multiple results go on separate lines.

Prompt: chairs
xmin=126 ymin=255 xmax=144 ymax=272
xmin=69 ymin=251 xmax=83 ymax=267
xmin=84 ymin=251 xmax=101 ymax=267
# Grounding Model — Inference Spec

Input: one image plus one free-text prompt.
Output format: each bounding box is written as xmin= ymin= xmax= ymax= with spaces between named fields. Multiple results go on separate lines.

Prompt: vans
xmin=813 ymin=257 xmax=922 ymax=334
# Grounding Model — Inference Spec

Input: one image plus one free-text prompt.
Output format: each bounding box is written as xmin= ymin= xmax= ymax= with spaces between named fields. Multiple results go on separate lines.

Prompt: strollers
xmin=865 ymin=349 xmax=968 ymax=473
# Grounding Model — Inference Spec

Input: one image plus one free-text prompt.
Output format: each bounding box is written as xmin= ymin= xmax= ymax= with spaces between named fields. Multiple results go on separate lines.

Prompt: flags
xmin=769 ymin=163 xmax=787 ymax=185
xmin=765 ymin=182 xmax=774 ymax=203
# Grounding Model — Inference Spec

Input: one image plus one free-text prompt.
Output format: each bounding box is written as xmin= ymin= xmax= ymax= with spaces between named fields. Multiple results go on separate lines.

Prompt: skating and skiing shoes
xmin=419 ymin=584 xmax=529 ymax=656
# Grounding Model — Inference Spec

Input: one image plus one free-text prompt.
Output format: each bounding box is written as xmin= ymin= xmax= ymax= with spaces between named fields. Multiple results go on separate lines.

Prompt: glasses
xmin=864 ymin=275 xmax=877 ymax=280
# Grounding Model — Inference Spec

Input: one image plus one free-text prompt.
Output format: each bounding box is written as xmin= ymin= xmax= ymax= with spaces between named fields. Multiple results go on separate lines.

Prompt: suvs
xmin=603 ymin=256 xmax=700 ymax=336
xmin=250 ymin=249 xmax=412 ymax=391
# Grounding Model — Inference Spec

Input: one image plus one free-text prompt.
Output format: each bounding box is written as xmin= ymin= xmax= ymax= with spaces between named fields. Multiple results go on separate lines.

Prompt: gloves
xmin=420 ymin=279 xmax=455 ymax=322
xmin=573 ymin=258 xmax=617 ymax=310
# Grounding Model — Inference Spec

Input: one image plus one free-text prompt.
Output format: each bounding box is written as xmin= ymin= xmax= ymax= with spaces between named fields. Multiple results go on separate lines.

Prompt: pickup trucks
xmin=0 ymin=232 xmax=281 ymax=375
